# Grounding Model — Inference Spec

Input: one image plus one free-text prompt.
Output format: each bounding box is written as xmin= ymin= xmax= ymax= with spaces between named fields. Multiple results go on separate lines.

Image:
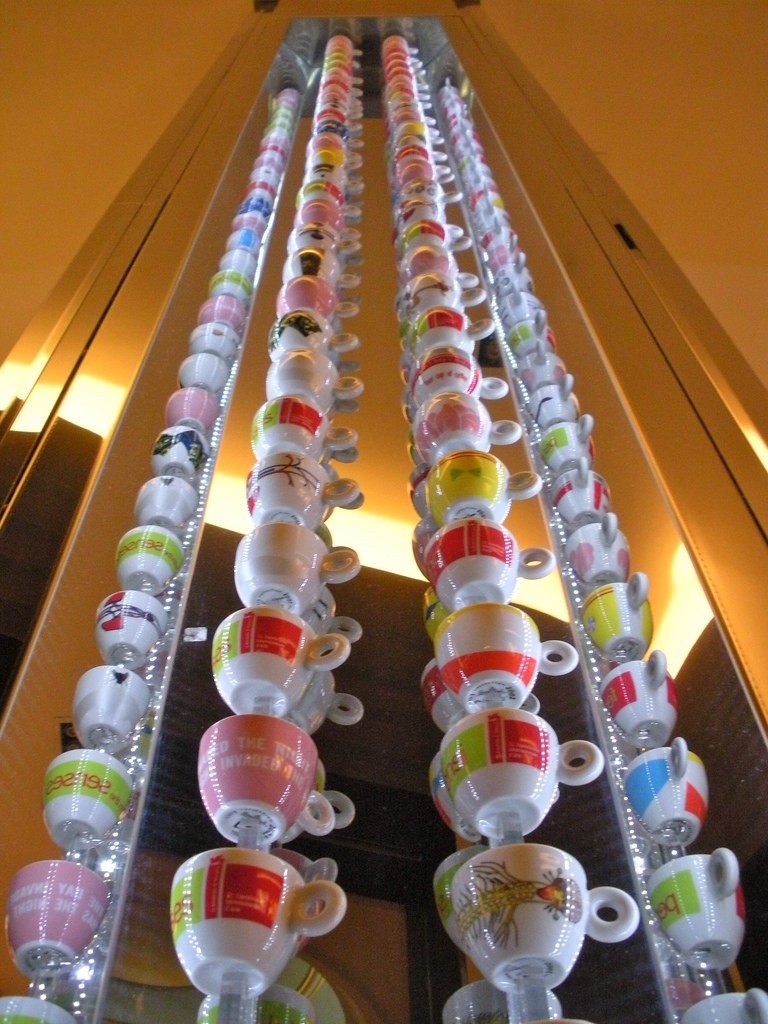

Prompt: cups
xmin=1 ymin=29 xmax=768 ymax=1023
